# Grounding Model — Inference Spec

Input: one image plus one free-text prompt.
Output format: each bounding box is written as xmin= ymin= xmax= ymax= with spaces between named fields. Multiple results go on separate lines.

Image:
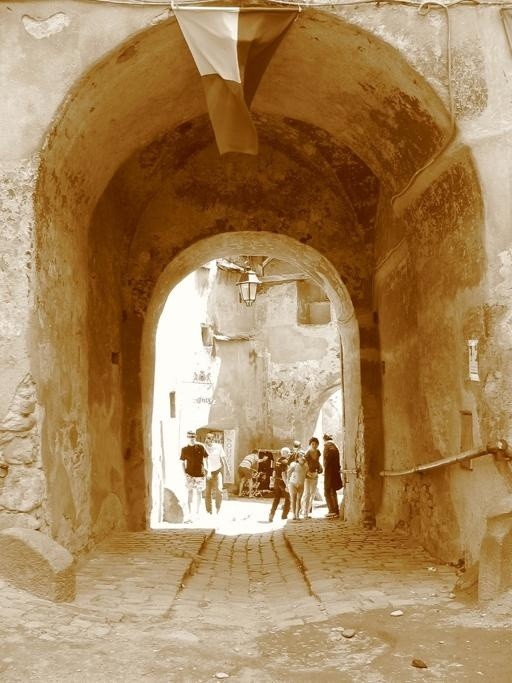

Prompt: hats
xmin=187 ymin=432 xmax=198 ymax=438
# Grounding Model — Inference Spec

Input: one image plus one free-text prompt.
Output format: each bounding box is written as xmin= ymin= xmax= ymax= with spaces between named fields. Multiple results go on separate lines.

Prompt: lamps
xmin=236 ymin=256 xmax=263 ymax=308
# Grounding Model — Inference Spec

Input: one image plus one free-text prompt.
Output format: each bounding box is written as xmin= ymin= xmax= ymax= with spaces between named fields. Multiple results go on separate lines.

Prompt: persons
xmin=267 ymin=437 xmax=323 ymax=523
xmin=203 ymin=434 xmax=233 ymax=516
xmin=179 ymin=431 xmax=211 ymax=526
xmin=236 ymin=449 xmax=270 ymax=499
xmin=323 ymin=433 xmax=344 ymax=521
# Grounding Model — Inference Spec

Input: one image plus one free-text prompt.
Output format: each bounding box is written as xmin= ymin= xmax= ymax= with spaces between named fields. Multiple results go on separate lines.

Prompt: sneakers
xmin=326 ymin=512 xmax=340 ymax=518
xmin=268 ymin=515 xmax=312 ymax=521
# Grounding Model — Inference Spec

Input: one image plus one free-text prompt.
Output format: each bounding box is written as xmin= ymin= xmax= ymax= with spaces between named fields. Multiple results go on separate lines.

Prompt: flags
xmin=170 ymin=6 xmax=303 ymax=157
